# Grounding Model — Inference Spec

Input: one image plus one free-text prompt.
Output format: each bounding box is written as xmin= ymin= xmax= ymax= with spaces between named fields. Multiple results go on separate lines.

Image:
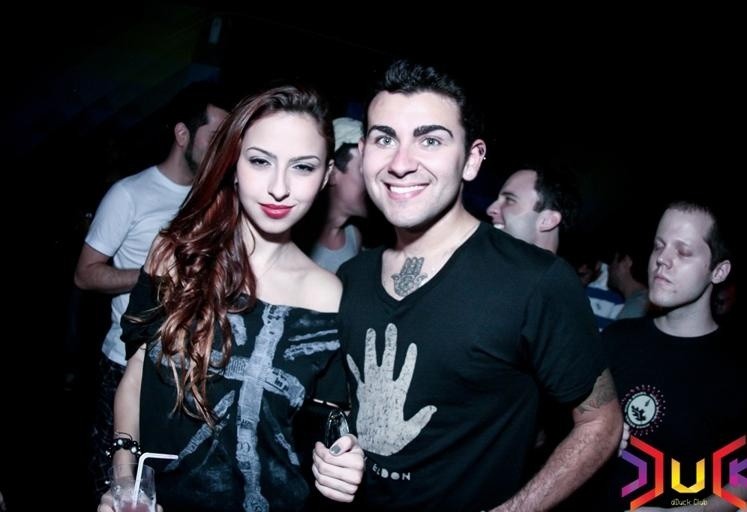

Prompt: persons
xmin=71 ymin=86 xmax=747 ymax=512
xmin=336 ymin=61 xmax=622 ymax=512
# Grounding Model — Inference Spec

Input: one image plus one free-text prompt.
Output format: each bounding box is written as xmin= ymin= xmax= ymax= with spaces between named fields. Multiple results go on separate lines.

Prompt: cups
xmin=107 ymin=463 xmax=156 ymax=512
